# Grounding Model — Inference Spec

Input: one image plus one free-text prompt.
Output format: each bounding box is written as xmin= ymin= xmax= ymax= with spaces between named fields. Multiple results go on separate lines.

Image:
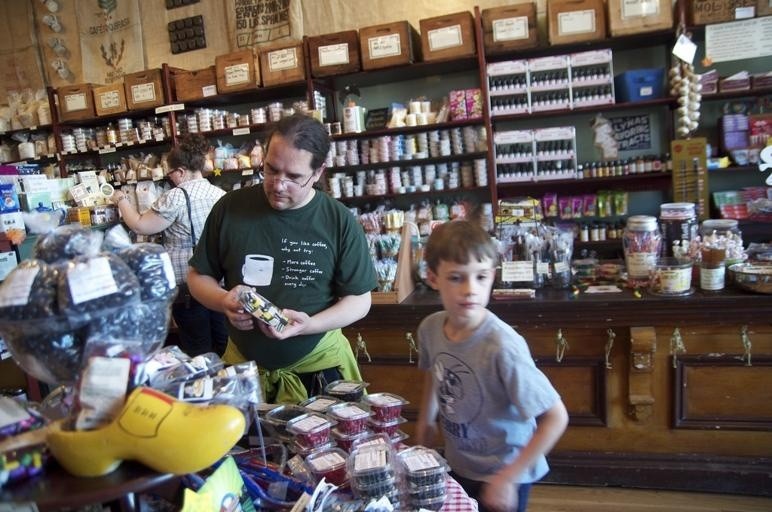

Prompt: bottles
xmin=622 ymin=160 xmax=629 ymax=175
xmin=532 ymin=92 xmax=569 ymax=107
xmin=583 ymin=161 xmax=590 ymax=178
xmin=573 ymin=67 xmax=610 ymax=82
xmin=538 ymin=160 xmax=574 ymax=175
xmin=644 ymin=155 xmax=652 ymax=173
xmin=666 ymin=153 xmax=672 ymax=171
xmin=497 ymin=162 xmax=534 ymax=178
xmin=599 ymin=222 xmax=605 ymax=241
xmin=497 ymin=143 xmax=531 ymax=159
xmin=596 ymin=161 xmax=603 ymax=177
xmin=699 ymin=245 xmax=725 ymax=293
xmin=699 ymin=219 xmax=742 ymax=240
xmin=580 ymin=221 xmax=588 ymax=243
xmin=637 ymin=155 xmax=645 ymax=174
xmin=657 ymin=202 xmax=699 ymax=268
xmin=603 ymin=161 xmax=609 ymax=177
xmin=615 ymin=160 xmax=622 ymax=176
xmin=106 ymin=123 xmax=117 ymax=146
xmin=590 ymin=221 xmax=600 ymax=241
xmin=590 ymin=161 xmax=596 ymax=177
xmin=491 ymin=77 xmax=526 ymax=90
xmin=661 ymin=153 xmax=666 ymax=172
xmin=578 ymin=164 xmax=583 ymax=179
xmin=622 ymin=214 xmax=663 ymax=281
xmin=573 ymin=88 xmax=611 ymax=101
xmin=653 ymin=156 xmax=661 ymax=172
xmin=629 ymin=156 xmax=636 ymax=175
xmin=609 ymin=161 xmax=615 ymax=176
xmin=537 ymin=139 xmax=573 ymax=156
xmin=531 ymin=72 xmax=567 ymax=87
xmin=492 ymin=98 xmax=527 ymax=111
xmin=343 ymin=100 xmax=366 ymax=133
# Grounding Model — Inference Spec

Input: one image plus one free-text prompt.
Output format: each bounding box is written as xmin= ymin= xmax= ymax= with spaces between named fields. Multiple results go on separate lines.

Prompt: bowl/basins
xmin=728 ymin=261 xmax=772 ymax=295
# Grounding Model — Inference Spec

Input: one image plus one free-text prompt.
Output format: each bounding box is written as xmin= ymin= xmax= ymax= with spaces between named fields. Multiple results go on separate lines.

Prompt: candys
xmin=681 ymin=240 xmax=689 ymax=255
xmin=709 ymin=230 xmax=717 ymax=243
xmin=672 ymin=240 xmax=681 ymax=257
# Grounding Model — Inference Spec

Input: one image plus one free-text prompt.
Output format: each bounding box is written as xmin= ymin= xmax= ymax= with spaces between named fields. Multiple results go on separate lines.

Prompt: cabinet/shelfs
xmin=0 ymin=87 xmax=60 ymax=181
xmin=307 ymin=7 xmax=496 ymax=240
xmin=166 ymin=36 xmax=319 ymax=192
xmin=37 ymin=440 xmax=479 ymax=512
xmin=683 ymin=2 xmax=772 ymax=230
xmin=475 ymin=0 xmax=680 ymax=247
xmin=48 ymin=62 xmax=171 ymax=184
xmin=343 ymin=284 xmax=772 ymax=493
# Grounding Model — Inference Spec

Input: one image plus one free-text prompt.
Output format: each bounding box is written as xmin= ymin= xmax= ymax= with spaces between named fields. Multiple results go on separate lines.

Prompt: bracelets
xmin=114 ymin=195 xmax=127 ymax=204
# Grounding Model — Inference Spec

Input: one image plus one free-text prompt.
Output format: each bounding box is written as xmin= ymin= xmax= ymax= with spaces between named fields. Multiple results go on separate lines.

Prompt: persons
xmin=413 ymin=220 xmax=566 ymax=512
xmin=187 ymin=112 xmax=379 ymax=399
xmin=112 ymin=133 xmax=229 ymax=358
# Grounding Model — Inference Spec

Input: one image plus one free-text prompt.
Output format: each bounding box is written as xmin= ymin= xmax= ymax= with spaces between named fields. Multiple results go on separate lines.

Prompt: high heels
xmin=45 ymin=384 xmax=247 ymax=479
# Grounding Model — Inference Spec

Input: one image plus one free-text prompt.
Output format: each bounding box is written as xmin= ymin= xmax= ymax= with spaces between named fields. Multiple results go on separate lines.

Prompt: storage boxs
xmin=482 ymin=2 xmax=537 ymax=54
xmin=419 ymin=11 xmax=475 ymax=63
xmin=260 ymin=40 xmax=306 ymax=87
xmin=359 ymin=20 xmax=421 ymax=70
xmin=608 ymin=0 xmax=674 ymax=37
xmin=614 ymin=68 xmax=663 ymax=102
xmin=547 ymin=0 xmax=606 ymax=45
xmin=215 ymin=48 xmax=261 ymax=93
xmin=57 ymin=83 xmax=105 ymax=121
xmin=124 ymin=68 xmax=165 ymax=111
xmin=309 ymin=30 xmax=360 ymax=77
xmin=689 ymin=0 xmax=756 ymax=25
xmin=175 ymin=65 xmax=217 ymax=102
xmin=92 ymin=81 xmax=127 ymax=117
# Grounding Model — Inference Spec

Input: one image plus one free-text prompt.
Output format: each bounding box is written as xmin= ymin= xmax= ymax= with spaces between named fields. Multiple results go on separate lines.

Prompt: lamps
xmin=41 ymin=0 xmax=59 ymax=12
xmin=50 ymin=58 xmax=69 ymax=79
xmin=42 ymin=13 xmax=61 ymax=32
xmin=45 ymin=37 xmax=66 ymax=56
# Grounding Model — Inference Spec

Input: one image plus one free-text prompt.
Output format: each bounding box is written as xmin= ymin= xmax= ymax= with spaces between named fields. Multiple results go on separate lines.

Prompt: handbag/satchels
xmin=155 ymin=188 xmax=206 ymax=320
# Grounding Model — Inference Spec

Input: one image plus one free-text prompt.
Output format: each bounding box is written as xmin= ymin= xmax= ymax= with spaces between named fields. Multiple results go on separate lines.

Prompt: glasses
xmin=255 ymin=159 xmax=321 ymax=191
xmin=166 ymin=166 xmax=188 ymax=177
